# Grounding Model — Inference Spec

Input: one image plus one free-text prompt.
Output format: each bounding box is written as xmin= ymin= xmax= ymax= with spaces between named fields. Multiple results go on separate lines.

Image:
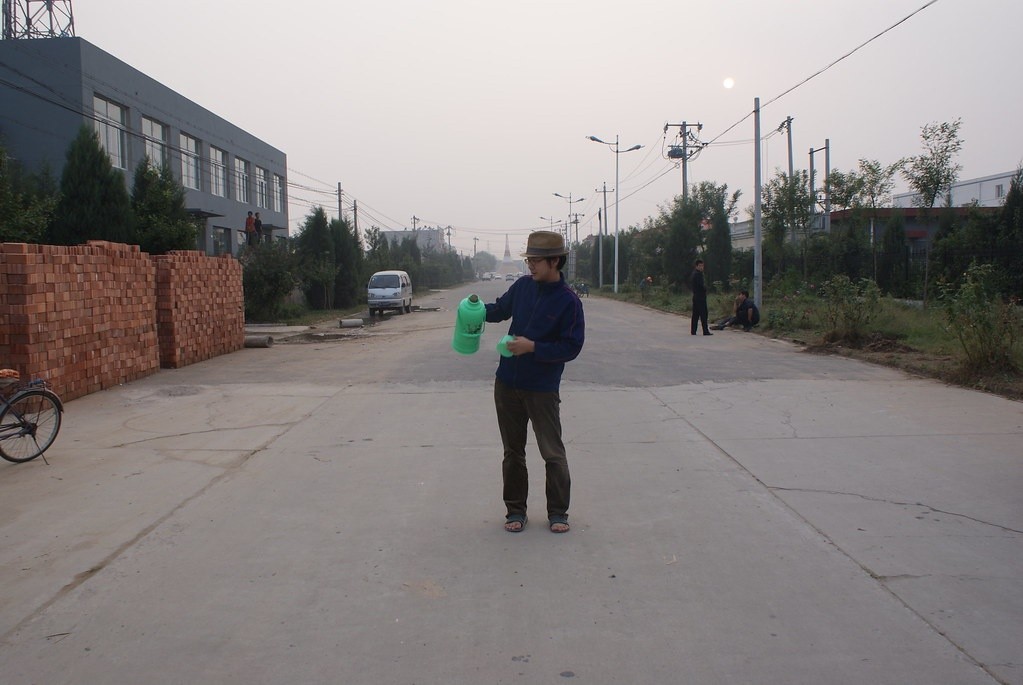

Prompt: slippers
xmin=505 ymin=513 xmax=528 ymax=531
xmin=549 ymin=515 xmax=569 ymax=533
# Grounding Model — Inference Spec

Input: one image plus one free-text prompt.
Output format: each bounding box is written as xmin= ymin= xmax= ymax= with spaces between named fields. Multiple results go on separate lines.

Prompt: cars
xmin=506 ymin=273 xmax=514 ymax=282
xmin=516 ymin=272 xmax=524 ymax=279
xmin=494 ymin=274 xmax=502 ymax=280
xmin=482 ymin=271 xmax=496 ymax=281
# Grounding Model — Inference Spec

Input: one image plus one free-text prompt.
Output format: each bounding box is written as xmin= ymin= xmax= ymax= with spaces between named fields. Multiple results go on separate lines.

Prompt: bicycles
xmin=0 ymin=369 xmax=65 ymax=465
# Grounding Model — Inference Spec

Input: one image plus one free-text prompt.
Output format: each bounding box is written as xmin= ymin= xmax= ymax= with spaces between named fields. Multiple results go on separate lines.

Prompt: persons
xmin=484 ymin=230 xmax=585 ymax=532
xmin=254 ymin=212 xmax=262 ymax=244
xmin=639 ymin=276 xmax=652 ymax=300
xmin=245 ymin=211 xmax=256 ymax=248
xmin=710 ymin=290 xmax=760 ymax=332
xmin=690 ymin=260 xmax=713 ymax=336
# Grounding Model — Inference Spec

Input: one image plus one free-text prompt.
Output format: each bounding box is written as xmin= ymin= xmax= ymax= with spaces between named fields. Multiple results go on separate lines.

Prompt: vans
xmin=366 ymin=270 xmax=412 ymax=316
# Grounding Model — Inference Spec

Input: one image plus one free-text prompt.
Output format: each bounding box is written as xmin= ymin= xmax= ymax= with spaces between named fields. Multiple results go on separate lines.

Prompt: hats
xmin=520 ymin=231 xmax=569 ymax=257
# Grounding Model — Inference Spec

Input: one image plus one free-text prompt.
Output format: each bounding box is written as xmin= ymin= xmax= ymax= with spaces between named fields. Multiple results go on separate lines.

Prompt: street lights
xmin=585 ymin=133 xmax=645 ymax=294
xmin=540 ymin=215 xmax=561 ymax=232
xmin=552 ymin=192 xmax=584 ymax=251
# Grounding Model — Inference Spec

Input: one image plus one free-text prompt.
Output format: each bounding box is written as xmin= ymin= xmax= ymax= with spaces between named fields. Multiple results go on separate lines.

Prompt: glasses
xmin=524 ymin=257 xmax=546 ymax=265
